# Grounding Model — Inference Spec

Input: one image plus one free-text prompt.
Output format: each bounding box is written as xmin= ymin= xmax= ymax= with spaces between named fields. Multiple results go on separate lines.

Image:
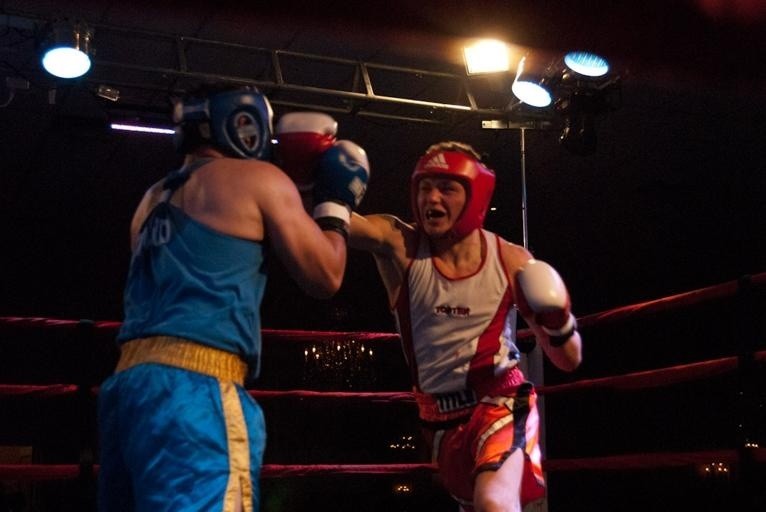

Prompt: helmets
xmin=410 ymin=141 xmax=496 ymax=248
xmin=170 ymin=85 xmax=274 ymax=159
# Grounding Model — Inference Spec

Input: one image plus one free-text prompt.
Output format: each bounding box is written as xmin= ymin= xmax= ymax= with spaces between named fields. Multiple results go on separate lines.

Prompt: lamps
xmin=38 ymin=11 xmax=93 ymax=78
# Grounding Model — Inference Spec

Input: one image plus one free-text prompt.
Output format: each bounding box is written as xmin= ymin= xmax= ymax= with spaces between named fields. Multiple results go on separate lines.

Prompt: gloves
xmin=275 ymin=112 xmax=370 ymax=243
xmin=514 ymin=259 xmax=577 ymax=344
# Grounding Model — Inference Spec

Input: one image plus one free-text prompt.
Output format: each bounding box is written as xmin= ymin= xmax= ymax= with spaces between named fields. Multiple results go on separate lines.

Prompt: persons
xmin=274 ymin=110 xmax=584 ymax=512
xmin=94 ymin=82 xmax=373 ymax=511
